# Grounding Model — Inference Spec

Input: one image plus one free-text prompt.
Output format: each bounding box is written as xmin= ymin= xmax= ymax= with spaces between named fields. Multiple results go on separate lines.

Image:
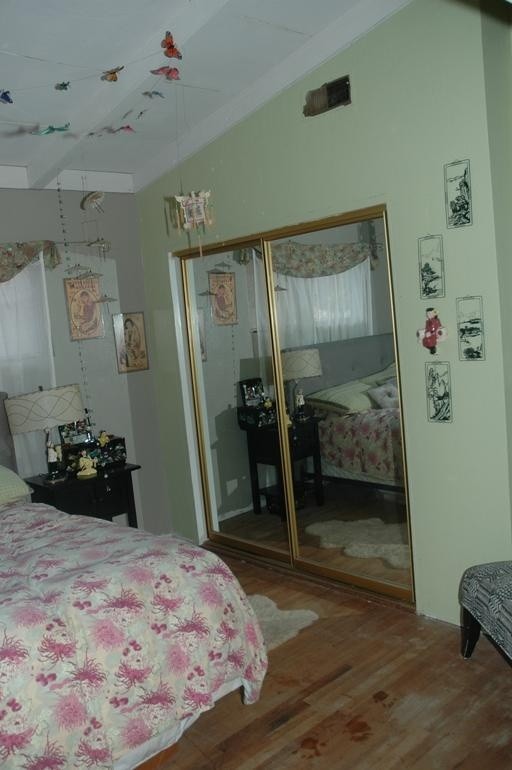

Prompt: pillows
xmin=305 ymin=361 xmax=399 ymax=415
xmin=0 ymin=464 xmax=34 ymax=506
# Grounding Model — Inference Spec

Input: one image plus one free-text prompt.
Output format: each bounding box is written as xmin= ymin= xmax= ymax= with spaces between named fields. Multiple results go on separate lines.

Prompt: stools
xmin=459 ymin=559 xmax=511 ymax=668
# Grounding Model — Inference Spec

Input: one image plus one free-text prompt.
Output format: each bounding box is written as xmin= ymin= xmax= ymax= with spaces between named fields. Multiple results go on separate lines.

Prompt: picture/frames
xmin=64 ymin=277 xmax=105 ymax=341
xmin=112 ymin=312 xmax=148 ymax=373
xmin=197 ymin=306 xmax=207 ymax=362
xmin=208 ymin=272 xmax=239 ymax=326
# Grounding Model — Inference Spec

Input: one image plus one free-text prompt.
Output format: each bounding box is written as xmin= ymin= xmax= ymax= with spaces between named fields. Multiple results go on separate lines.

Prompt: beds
xmin=0 ymin=390 xmax=269 ymax=770
xmin=281 ymin=332 xmax=406 ymax=505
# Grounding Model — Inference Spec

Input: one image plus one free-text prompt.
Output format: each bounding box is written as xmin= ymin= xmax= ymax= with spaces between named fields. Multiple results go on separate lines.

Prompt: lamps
xmin=4 ymin=383 xmax=84 ymax=484
xmin=281 ymin=349 xmax=321 ymax=422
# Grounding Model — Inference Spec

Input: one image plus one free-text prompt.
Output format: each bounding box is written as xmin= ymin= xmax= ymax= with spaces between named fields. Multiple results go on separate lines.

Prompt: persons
xmin=215 ymin=284 xmax=234 ymax=319
xmin=44 ymin=427 xmax=60 ymax=474
xmin=119 ymin=319 xmax=146 ymax=369
xmin=422 ymin=308 xmax=442 ymax=354
xmin=71 ymin=293 xmax=98 ymax=334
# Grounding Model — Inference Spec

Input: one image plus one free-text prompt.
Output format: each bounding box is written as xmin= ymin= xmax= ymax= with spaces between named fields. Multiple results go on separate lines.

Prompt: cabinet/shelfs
xmin=247 ymin=413 xmax=324 ymax=521
xmin=23 ymin=455 xmax=141 ymax=530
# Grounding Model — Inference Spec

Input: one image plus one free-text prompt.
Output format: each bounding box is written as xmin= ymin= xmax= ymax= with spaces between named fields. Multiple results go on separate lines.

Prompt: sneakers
xmin=431 ymin=347 xmax=437 ymax=354
